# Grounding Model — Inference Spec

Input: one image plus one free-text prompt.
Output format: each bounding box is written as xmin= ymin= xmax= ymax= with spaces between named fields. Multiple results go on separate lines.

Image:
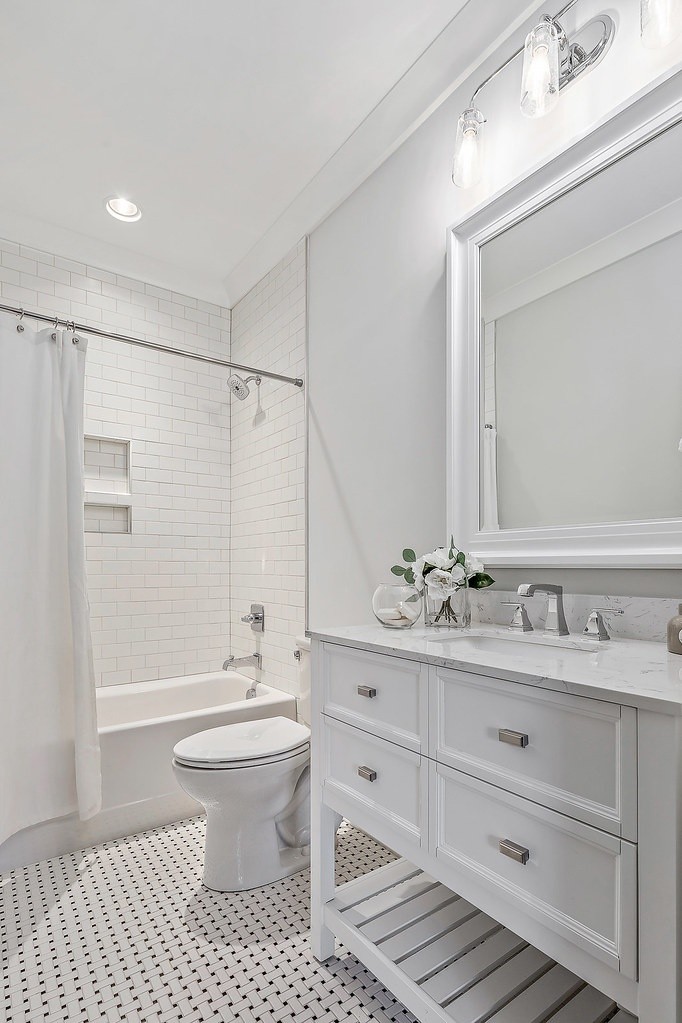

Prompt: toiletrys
xmin=665 ymin=603 xmax=682 ymax=655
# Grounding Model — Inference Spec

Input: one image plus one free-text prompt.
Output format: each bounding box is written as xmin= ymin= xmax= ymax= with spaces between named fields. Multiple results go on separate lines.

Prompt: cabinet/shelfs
xmin=309 ymin=638 xmax=682 ymax=1023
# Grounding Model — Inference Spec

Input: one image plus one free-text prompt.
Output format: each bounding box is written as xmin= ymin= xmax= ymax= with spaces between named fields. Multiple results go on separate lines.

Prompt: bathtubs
xmin=1 ymin=668 xmax=299 ymax=878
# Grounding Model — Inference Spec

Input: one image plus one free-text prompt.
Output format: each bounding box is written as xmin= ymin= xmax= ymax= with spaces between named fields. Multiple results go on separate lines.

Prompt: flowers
xmin=389 ymin=534 xmax=496 ymax=624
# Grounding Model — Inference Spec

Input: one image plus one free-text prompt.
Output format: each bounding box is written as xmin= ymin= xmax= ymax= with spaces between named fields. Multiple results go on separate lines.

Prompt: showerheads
xmin=226 ymin=373 xmax=261 ymax=402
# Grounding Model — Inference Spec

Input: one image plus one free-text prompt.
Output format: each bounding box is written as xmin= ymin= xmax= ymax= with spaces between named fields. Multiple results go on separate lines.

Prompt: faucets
xmin=516 ymin=582 xmax=571 ymax=637
xmin=221 ymin=652 xmax=263 ymax=672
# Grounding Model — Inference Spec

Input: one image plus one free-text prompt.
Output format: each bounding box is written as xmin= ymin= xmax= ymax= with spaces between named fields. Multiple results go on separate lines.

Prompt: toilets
xmin=170 ymin=635 xmax=340 ymax=892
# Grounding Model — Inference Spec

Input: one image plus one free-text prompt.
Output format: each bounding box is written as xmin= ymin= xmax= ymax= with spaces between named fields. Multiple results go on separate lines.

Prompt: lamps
xmin=451 ymin=0 xmax=678 ymax=191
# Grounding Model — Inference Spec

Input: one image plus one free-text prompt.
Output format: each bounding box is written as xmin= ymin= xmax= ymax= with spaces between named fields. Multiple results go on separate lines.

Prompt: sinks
xmin=423 ymin=628 xmax=606 ymax=665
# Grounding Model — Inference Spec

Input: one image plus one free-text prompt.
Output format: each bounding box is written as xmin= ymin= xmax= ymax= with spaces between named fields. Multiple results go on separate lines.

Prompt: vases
xmin=424 ymin=584 xmax=472 ymax=630
xmin=372 ymin=583 xmax=423 ymax=630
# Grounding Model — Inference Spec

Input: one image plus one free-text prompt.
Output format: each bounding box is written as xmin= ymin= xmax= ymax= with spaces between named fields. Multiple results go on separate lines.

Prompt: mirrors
xmin=476 ymin=119 xmax=681 ymax=531
